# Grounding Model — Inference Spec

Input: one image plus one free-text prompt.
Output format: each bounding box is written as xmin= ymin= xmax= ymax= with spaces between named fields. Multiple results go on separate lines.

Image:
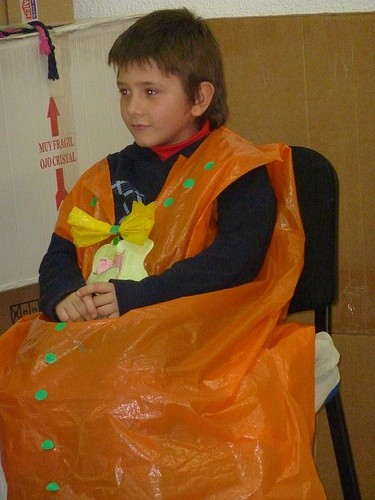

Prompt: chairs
xmin=283 ymin=144 xmax=361 ymax=500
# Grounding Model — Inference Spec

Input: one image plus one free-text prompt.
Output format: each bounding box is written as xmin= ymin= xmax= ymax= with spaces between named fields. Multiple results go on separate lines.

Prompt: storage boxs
xmin=0 ymin=0 xmax=74 ymax=30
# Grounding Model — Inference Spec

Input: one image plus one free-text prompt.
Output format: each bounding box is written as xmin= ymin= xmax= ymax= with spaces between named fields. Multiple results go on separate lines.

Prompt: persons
xmin=38 ymin=7 xmax=280 ymax=322
